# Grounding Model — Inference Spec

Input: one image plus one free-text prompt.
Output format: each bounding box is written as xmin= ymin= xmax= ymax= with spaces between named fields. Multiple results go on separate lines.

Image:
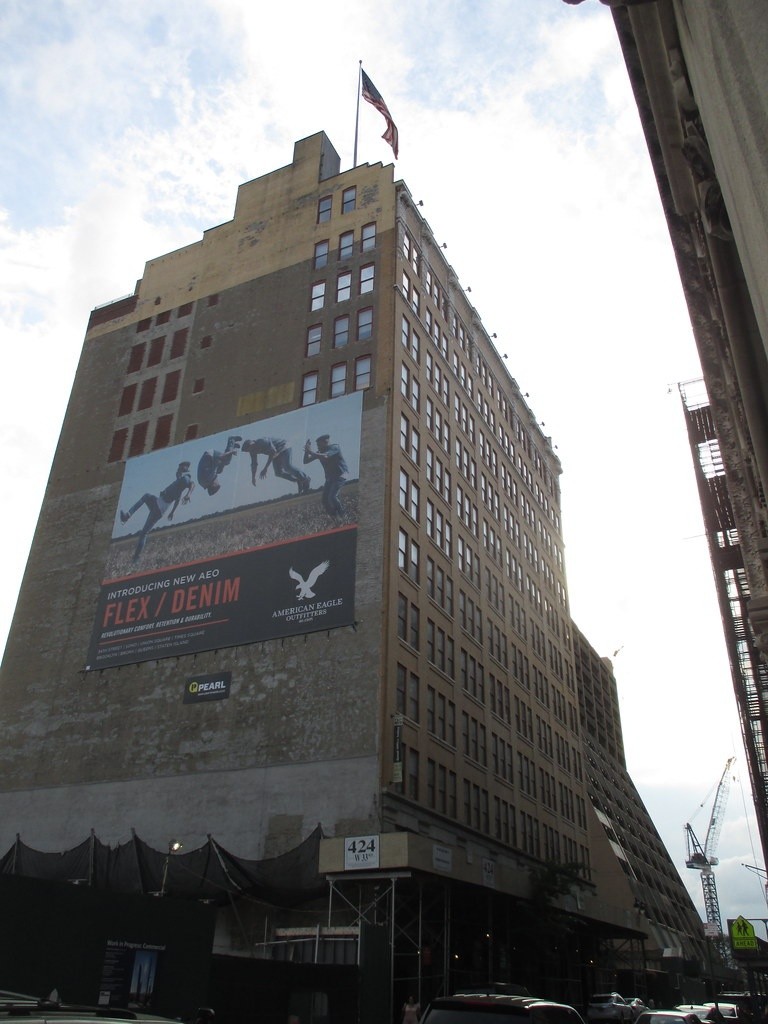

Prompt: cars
xmin=624 ymin=997 xmax=649 ymax=1019
xmin=419 ymin=993 xmax=585 ymax=1024
xmin=635 ymin=1009 xmax=702 ymax=1024
xmin=672 ymin=1005 xmax=729 ymax=1024
xmin=703 ymin=1002 xmax=752 ymax=1024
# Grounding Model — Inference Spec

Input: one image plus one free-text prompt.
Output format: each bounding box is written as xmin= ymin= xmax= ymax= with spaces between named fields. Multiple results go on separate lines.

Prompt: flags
xmin=362 ymin=69 xmax=399 ymax=160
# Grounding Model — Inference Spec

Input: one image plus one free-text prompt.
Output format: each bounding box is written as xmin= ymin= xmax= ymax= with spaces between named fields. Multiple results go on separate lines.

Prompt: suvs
xmin=587 ymin=992 xmax=635 ymax=1024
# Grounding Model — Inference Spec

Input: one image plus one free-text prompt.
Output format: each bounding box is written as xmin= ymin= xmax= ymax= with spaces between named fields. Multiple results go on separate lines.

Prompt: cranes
xmin=686 ymin=754 xmax=735 ymax=966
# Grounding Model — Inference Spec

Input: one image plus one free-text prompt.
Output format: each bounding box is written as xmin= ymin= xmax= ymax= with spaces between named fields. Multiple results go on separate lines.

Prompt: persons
xmin=402 ymin=993 xmax=420 ymax=1024
xmin=303 ymin=435 xmax=349 ymax=520
xmin=121 ymin=462 xmax=195 ymax=563
xmin=241 ymin=438 xmax=311 ymax=494
xmin=197 ymin=436 xmax=242 ymax=496
